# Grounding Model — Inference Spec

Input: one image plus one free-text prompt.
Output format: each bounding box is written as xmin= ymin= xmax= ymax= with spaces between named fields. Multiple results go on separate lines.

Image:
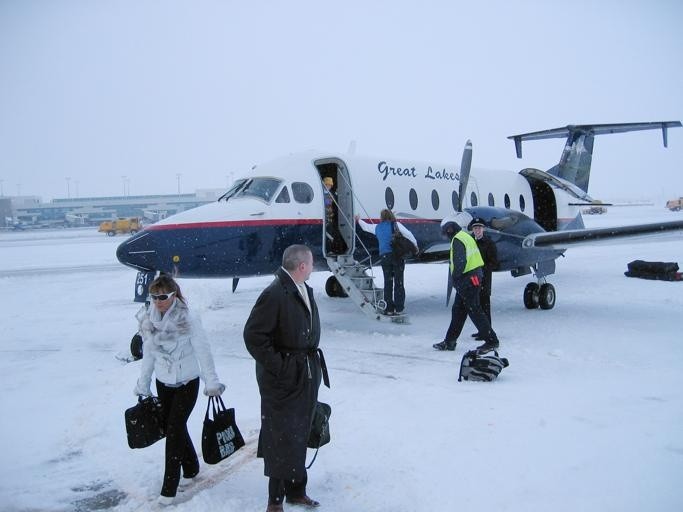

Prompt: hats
xmin=324 ymin=177 xmax=333 ymax=185
xmin=470 ymin=218 xmax=485 ymax=227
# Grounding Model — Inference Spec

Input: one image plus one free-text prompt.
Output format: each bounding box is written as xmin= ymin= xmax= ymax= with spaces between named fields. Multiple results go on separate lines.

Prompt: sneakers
xmin=157 ymin=494 xmax=175 ymax=505
xmin=265 ymin=501 xmax=284 ymax=512
xmin=473 ymin=332 xmax=499 ymax=354
xmin=382 ymin=310 xmax=404 ymax=316
xmin=286 ymin=496 xmax=320 ymax=507
xmin=179 ymin=476 xmax=192 ymax=487
xmin=433 ymin=341 xmax=457 ymax=350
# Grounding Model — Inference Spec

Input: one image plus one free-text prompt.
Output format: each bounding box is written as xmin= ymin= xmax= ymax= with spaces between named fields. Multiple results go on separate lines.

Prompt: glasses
xmin=152 ymin=291 xmax=175 ymax=302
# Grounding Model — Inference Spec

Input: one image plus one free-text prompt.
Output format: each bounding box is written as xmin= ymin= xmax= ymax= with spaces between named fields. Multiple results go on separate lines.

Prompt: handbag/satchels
xmin=327 ymin=224 xmax=347 ymax=254
xmin=308 ymin=402 xmax=331 ymax=449
xmin=390 ymin=233 xmax=420 ymax=258
xmin=202 ymin=395 xmax=246 ymax=464
xmin=124 ymin=396 xmax=167 ymax=448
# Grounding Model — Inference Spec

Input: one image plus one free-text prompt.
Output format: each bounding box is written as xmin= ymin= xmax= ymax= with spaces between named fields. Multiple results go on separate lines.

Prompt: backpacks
xmin=459 ymin=349 xmax=509 ymax=381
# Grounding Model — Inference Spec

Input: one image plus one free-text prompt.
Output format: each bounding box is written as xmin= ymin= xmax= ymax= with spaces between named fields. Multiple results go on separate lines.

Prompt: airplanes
xmin=116 ymin=120 xmax=683 ymax=308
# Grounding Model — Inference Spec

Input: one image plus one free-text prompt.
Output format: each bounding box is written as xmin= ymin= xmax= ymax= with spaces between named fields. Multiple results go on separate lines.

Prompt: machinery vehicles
xmin=98 ymin=216 xmax=144 ymax=236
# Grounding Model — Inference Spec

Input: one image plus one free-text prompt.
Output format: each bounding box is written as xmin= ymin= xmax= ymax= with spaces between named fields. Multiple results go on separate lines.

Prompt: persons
xmin=134 ymin=275 xmax=226 ymax=504
xmin=243 ymin=245 xmax=330 ymax=512
xmin=323 ymin=177 xmax=335 ymax=255
xmin=433 ymin=221 xmax=499 ymax=353
xmin=470 ymin=218 xmax=497 ymax=341
xmin=353 ymin=209 xmax=419 ymax=316
